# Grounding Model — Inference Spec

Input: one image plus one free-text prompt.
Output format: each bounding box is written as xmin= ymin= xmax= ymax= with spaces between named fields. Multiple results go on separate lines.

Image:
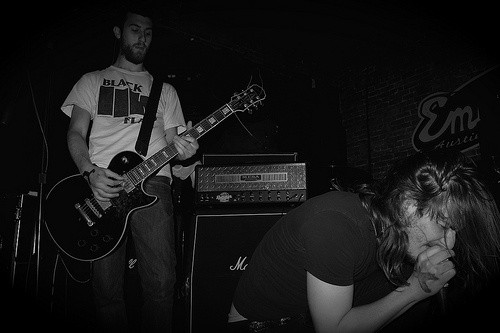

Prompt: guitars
xmin=43 ymin=83 xmax=267 ymax=263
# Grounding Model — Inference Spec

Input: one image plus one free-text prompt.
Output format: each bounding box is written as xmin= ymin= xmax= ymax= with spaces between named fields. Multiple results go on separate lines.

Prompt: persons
xmin=227 ymin=153 xmax=500 ymax=333
xmin=61 ymin=8 xmax=199 ymax=333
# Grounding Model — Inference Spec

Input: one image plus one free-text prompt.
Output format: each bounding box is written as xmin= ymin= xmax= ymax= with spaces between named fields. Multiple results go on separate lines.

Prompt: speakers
xmin=188 ymin=208 xmax=290 ymax=333
